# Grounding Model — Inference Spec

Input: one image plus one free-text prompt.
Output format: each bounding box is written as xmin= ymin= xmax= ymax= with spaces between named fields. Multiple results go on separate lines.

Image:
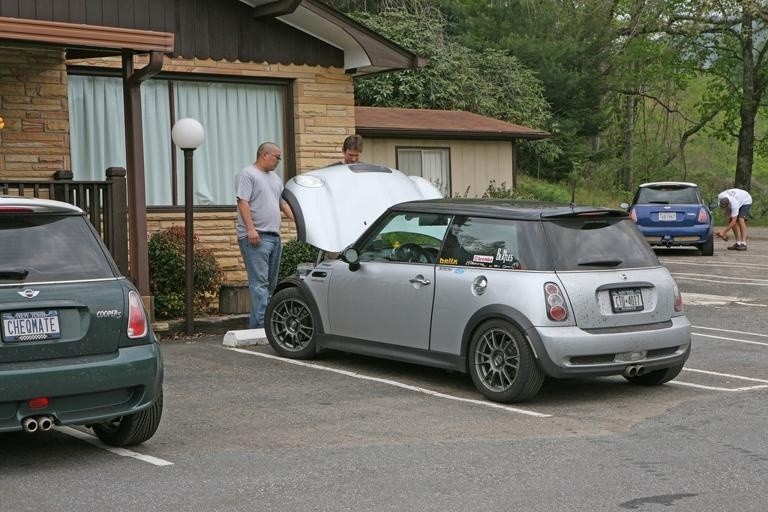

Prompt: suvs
xmin=618 ymin=179 xmax=721 ymax=259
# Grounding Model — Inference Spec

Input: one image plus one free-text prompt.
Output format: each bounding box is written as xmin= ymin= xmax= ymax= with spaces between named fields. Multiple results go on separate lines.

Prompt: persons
xmin=323 ymin=134 xmax=368 ymax=259
xmin=233 ymin=142 xmax=295 ymax=328
xmin=716 ymin=188 xmax=752 ymax=251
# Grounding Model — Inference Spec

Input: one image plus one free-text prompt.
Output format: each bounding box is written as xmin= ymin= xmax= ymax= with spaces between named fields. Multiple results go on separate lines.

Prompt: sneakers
xmin=727 ymin=243 xmax=747 ymax=250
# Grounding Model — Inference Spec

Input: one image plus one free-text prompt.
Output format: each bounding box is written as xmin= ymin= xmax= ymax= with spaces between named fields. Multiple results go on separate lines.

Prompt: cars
xmin=261 ymin=161 xmax=695 ymax=409
xmin=0 ymin=190 xmax=166 ymax=452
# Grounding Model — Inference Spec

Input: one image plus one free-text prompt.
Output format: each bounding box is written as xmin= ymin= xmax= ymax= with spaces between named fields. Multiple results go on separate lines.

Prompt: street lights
xmin=172 ymin=117 xmax=205 ymax=342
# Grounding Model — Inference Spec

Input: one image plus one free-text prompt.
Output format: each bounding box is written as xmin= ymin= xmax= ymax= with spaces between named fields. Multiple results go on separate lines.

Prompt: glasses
xmin=269 ymin=151 xmax=281 ymax=161
